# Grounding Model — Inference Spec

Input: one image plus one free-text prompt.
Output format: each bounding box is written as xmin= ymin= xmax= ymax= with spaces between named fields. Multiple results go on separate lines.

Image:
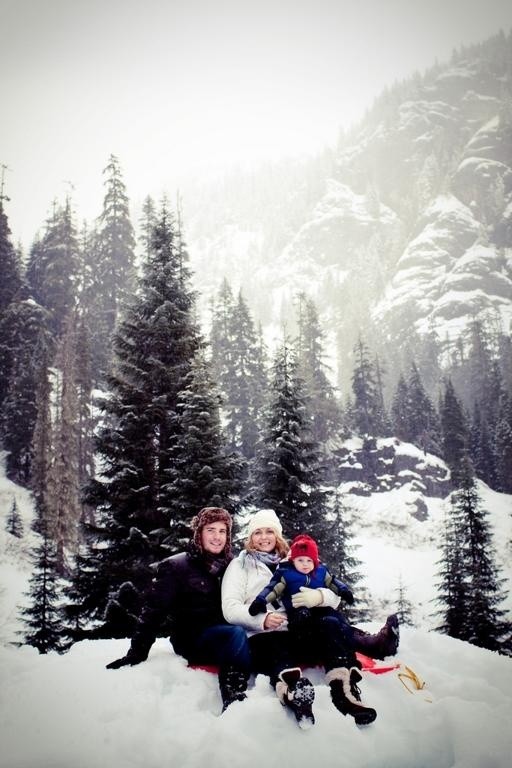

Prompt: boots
xmin=218 ymin=666 xmax=250 ymax=713
xmin=344 ymin=615 xmax=399 ymax=658
xmin=276 ymin=667 xmax=314 ymax=726
xmin=324 ymin=666 xmax=376 ymax=724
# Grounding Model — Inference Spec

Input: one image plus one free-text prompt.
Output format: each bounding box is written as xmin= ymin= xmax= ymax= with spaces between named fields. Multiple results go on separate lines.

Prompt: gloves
xmin=291 ymin=586 xmax=323 ymax=609
xmin=249 ymin=598 xmax=267 ymax=616
xmin=340 ymin=588 xmax=354 ymax=605
xmin=107 ymin=640 xmax=152 ymax=669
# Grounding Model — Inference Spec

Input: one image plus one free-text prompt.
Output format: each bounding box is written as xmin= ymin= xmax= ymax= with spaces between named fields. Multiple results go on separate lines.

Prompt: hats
xmin=190 ymin=507 xmax=233 ymax=560
xmin=288 ymin=534 xmax=320 ymax=570
xmin=248 ymin=509 xmax=282 ymax=538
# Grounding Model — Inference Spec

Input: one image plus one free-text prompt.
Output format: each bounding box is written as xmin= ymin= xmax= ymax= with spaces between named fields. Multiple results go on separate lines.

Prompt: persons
xmin=250 ymin=535 xmax=361 ymax=669
xmin=106 ymin=507 xmax=399 ymax=712
xmin=220 ymin=509 xmax=376 ymax=724
